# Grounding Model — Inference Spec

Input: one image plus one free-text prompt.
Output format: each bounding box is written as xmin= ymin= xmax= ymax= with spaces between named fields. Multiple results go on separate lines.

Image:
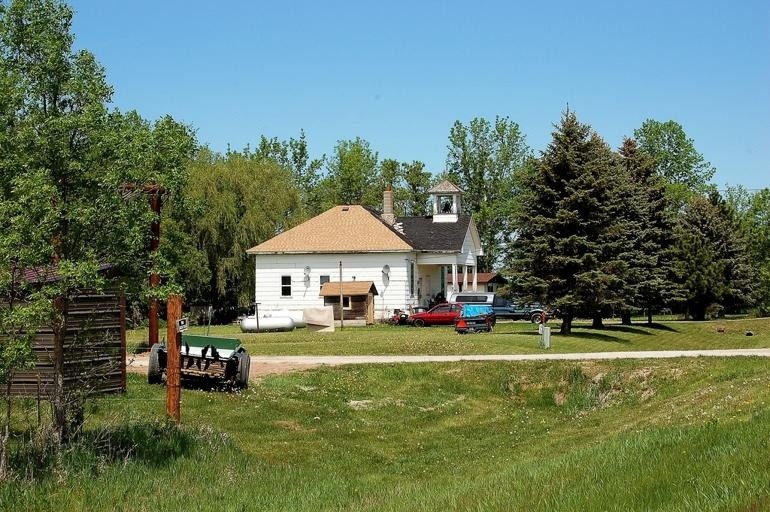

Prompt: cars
xmin=406 ymin=292 xmax=556 ymax=333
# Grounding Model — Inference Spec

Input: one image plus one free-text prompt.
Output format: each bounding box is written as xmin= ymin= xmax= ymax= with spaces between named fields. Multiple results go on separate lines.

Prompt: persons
xmin=444 ymin=201 xmax=451 ymax=213
